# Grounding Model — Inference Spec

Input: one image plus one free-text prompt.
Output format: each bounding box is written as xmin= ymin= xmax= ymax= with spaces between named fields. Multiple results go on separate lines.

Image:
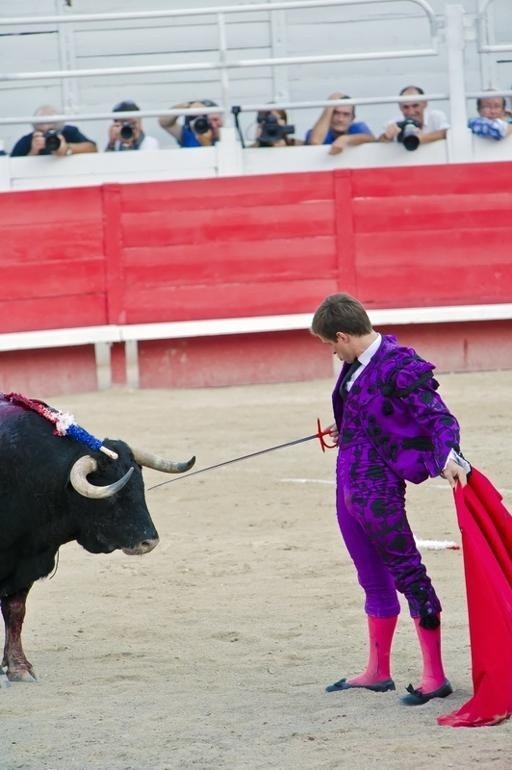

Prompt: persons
xmin=159 ymin=99 xmax=224 ymax=147
xmin=104 ymin=101 xmax=159 ymax=152
xmin=311 ymin=292 xmax=473 ymax=705
xmin=378 ymin=86 xmax=450 ymax=144
xmin=10 ymin=103 xmax=97 ymax=157
xmin=304 ymin=93 xmax=376 ymax=155
xmin=246 ymin=102 xmax=304 ymax=148
xmin=467 ymin=88 xmax=512 ymax=141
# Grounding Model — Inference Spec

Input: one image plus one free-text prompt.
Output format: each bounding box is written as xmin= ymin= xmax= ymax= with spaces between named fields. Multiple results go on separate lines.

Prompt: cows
xmin=0 ymin=392 xmax=197 ymax=683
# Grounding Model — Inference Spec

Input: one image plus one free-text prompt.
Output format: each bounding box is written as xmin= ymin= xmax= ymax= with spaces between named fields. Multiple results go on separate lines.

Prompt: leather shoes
xmin=325 ymin=678 xmax=396 ymax=692
xmin=401 ymin=679 xmax=452 ymax=705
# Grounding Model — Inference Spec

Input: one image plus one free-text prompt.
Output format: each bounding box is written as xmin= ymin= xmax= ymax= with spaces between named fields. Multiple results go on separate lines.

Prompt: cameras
xmin=186 ymin=115 xmax=208 ymax=133
xmin=397 ymin=117 xmax=422 ymax=151
xmin=258 ymin=112 xmax=295 ymax=144
xmin=120 ymin=122 xmax=133 ymax=139
xmin=45 ymin=129 xmax=60 ymax=151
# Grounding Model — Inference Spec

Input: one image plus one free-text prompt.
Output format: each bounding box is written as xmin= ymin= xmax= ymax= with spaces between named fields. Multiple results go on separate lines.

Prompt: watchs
xmin=64 ymin=142 xmax=72 ymax=156
